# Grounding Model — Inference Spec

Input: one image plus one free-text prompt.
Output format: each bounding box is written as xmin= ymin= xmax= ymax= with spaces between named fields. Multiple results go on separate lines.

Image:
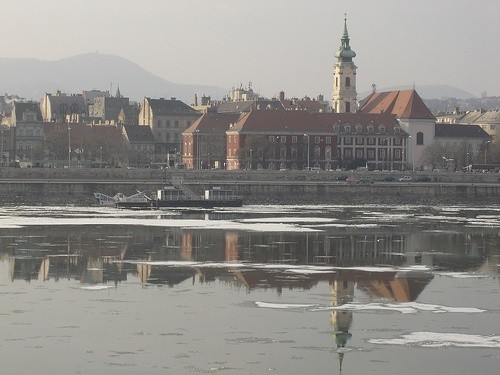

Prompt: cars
xmin=399 ymin=176 xmax=412 ymax=182
xmin=382 ymin=176 xmax=395 ymax=182
xmin=336 ymin=175 xmax=349 ymax=181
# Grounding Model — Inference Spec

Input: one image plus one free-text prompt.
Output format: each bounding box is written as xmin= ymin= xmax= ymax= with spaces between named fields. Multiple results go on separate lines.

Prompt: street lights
xmin=195 ymin=129 xmax=200 ymax=170
xmin=304 ymin=134 xmax=310 ymax=171
xmin=409 ymin=135 xmax=415 ymax=172
xmin=484 ymin=141 xmax=490 ymax=165
xmin=271 ymin=136 xmax=280 ymax=169
xmin=0 ymin=129 xmax=4 ymax=166
xmin=466 ymin=152 xmax=470 ymax=171
xmin=68 ymin=128 xmax=72 ymax=168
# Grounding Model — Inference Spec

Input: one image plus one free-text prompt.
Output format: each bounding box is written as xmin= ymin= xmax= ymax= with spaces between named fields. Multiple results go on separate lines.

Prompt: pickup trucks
xmin=357 ymin=176 xmax=374 ymax=184
